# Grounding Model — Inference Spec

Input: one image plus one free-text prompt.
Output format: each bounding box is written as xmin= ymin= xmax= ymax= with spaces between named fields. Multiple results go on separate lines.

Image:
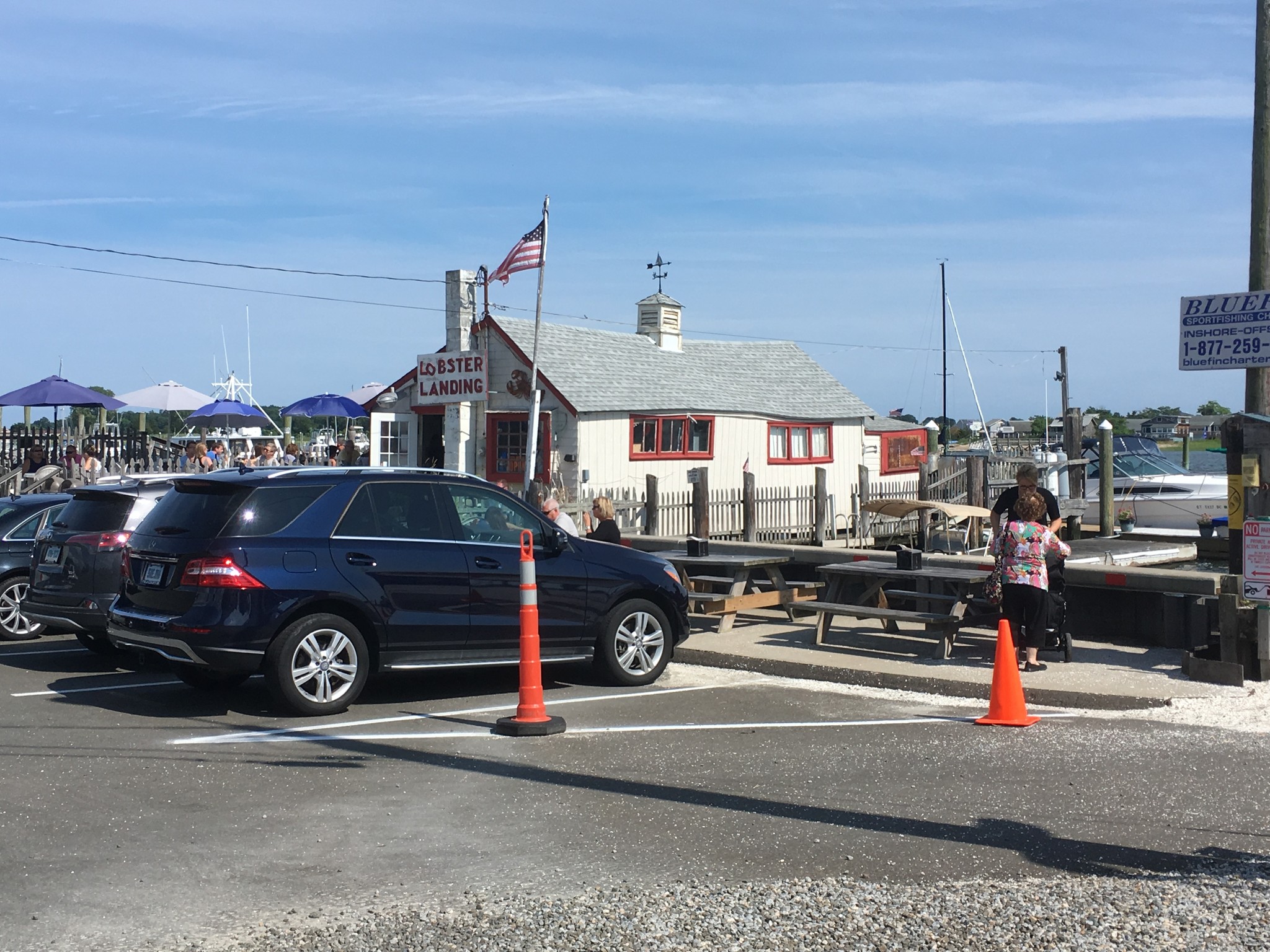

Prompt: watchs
xmin=584 ymin=526 xmax=591 ymax=530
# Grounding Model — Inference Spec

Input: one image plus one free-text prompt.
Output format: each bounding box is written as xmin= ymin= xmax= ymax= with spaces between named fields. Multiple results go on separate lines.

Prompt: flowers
xmin=1197 ymin=512 xmax=1215 ymax=524
xmin=1117 ymin=506 xmax=1135 ymax=521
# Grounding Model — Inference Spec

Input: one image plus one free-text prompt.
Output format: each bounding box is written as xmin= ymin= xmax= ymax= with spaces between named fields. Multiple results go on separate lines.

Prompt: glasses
xmin=593 ymin=505 xmax=599 ymax=510
xmin=337 ymin=444 xmax=343 ymax=448
xmin=264 ymin=448 xmax=274 ymax=453
xmin=205 ymin=449 xmax=208 ymax=451
xmin=66 ymin=451 xmax=75 ymax=454
xmin=1018 ymin=480 xmax=1038 ymax=490
xmin=237 ymin=458 xmax=245 ymax=460
xmin=542 ymin=507 xmax=557 ymax=515
xmin=188 ymin=446 xmax=196 ymax=449
xmin=32 ymin=449 xmax=43 ymax=452
xmin=335 ymin=452 xmax=339 ymax=457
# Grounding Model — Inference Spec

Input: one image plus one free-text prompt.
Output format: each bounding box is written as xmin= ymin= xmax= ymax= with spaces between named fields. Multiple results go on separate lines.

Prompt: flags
xmin=488 ymin=220 xmax=543 ymax=287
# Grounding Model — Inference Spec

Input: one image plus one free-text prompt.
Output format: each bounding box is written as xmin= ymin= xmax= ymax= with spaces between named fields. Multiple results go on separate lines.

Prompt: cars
xmin=98 ymin=464 xmax=695 ymax=715
xmin=0 ymin=494 xmax=85 ymax=643
xmin=24 ymin=469 xmax=201 ymax=652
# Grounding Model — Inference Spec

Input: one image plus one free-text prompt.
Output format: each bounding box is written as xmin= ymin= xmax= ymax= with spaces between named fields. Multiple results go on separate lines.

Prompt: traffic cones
xmin=970 ymin=615 xmax=1042 ymax=726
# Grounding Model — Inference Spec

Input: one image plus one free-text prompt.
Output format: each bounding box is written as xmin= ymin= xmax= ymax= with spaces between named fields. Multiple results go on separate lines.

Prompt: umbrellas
xmin=183 ymin=398 xmax=271 ymax=467
xmin=0 ymin=375 xmax=128 ymax=461
xmin=278 ymin=392 xmax=370 ymax=460
xmin=343 ymin=382 xmax=389 ymax=404
xmin=112 ymin=380 xmax=215 ymax=461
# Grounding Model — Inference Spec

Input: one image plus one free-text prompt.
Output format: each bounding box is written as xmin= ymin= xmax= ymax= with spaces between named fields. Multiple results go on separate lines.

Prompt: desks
xmin=812 ymin=559 xmax=997 ymax=658
xmin=643 ymin=551 xmax=790 ymax=634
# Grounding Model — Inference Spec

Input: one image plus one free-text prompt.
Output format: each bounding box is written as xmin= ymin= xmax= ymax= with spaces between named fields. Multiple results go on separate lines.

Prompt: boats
xmin=1065 ymin=434 xmax=1233 ymax=533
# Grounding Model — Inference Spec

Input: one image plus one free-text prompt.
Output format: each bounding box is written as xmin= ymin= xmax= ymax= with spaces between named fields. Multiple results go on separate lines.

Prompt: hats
xmin=325 ymin=445 xmax=338 ymax=458
xmin=237 ymin=452 xmax=247 ymax=459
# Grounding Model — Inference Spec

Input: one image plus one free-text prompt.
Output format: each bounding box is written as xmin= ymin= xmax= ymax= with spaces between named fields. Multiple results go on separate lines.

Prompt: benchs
xmin=686 ymin=575 xmax=1026 ymax=621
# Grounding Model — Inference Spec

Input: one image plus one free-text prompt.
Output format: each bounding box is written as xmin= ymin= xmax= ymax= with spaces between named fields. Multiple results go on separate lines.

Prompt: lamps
xmin=564 ymin=453 xmax=576 ymax=462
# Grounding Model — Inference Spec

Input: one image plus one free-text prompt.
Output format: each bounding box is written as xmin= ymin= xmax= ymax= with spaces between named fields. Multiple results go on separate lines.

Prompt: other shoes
xmin=1024 ymin=662 xmax=1047 ymax=672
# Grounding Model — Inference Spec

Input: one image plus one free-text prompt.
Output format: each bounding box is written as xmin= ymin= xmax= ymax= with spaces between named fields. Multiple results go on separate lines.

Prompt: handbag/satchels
xmin=981 ymin=568 xmax=1003 ymax=606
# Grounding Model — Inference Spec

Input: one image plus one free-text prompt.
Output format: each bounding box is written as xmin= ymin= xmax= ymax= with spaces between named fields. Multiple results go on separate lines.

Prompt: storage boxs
xmin=633 ymin=443 xmax=641 ymax=453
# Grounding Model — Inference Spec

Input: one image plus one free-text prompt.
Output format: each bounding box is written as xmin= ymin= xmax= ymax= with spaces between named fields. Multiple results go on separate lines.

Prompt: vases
xmin=1119 ymin=519 xmax=1136 ymax=533
xmin=1199 ymin=523 xmax=1215 ymax=536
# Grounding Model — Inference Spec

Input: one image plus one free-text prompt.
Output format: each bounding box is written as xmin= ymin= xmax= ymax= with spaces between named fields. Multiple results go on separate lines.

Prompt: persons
xmin=477 ymin=506 xmax=509 ymax=532
xmin=541 ymin=499 xmax=578 ymax=537
xmin=180 ymin=440 xmax=360 ymax=473
xmin=991 ymin=464 xmax=1062 ymax=540
xmin=990 ymin=492 xmax=1070 ymax=672
xmin=22 ymin=445 xmax=108 ymax=474
xmin=583 ymin=497 xmax=621 ymax=545
xmin=494 ymin=479 xmax=508 ymax=491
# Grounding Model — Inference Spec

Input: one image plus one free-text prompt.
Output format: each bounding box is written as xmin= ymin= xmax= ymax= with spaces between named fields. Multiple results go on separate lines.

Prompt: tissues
xmin=896 ymin=543 xmax=922 ymax=570
